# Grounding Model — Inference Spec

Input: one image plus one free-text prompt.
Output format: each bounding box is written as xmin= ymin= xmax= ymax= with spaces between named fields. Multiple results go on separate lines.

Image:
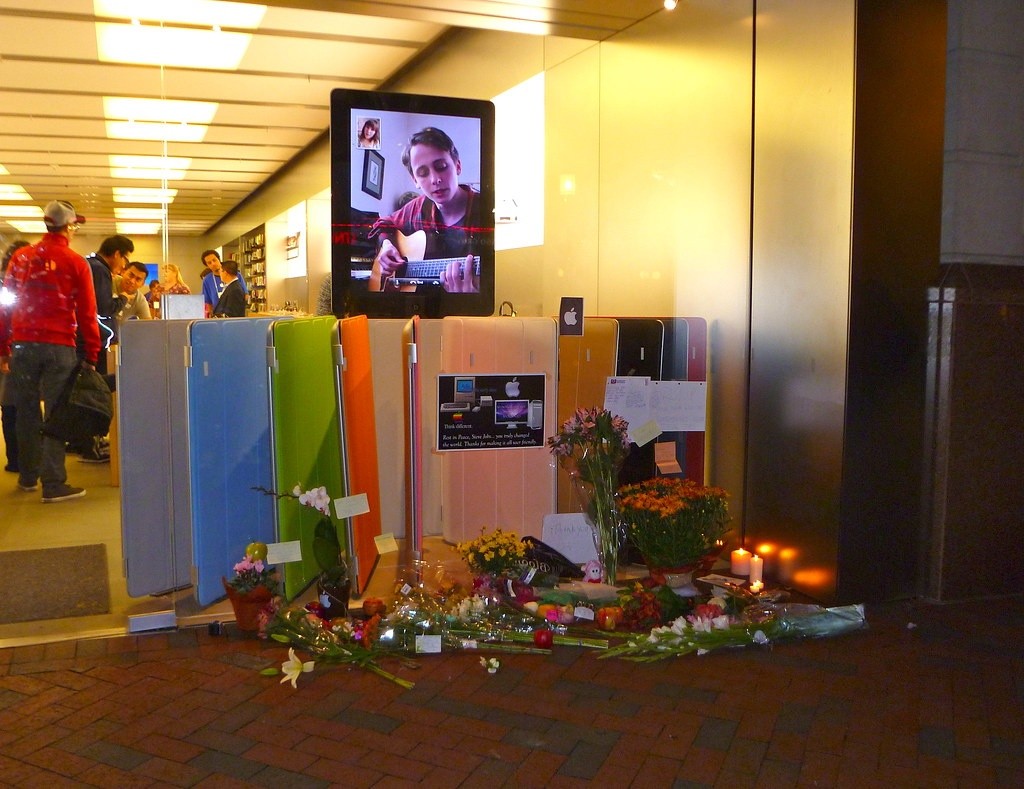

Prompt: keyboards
xmin=440 ymin=402 xmax=470 ymax=412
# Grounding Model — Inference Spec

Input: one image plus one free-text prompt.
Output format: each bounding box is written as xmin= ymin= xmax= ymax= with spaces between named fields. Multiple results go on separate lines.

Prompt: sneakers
xmin=40 ymin=483 xmax=87 ymax=504
xmin=75 ymin=449 xmax=111 ymax=463
xmin=16 ymin=476 xmax=39 ymax=492
xmin=98 ymin=439 xmax=110 ymax=451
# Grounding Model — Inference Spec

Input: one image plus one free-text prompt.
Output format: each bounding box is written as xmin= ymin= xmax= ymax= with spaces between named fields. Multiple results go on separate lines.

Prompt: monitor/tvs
xmin=327 ymin=89 xmax=496 ymax=321
xmin=495 ymin=400 xmax=529 ymax=429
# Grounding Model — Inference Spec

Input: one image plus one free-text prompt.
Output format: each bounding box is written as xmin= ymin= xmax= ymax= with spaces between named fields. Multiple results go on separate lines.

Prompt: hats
xmin=42 ymin=199 xmax=86 ymax=227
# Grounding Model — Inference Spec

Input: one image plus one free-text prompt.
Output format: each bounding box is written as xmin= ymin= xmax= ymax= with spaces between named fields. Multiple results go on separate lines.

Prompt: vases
xmin=221 ymin=576 xmax=272 ymax=630
xmin=316 ymin=574 xmax=351 ymax=619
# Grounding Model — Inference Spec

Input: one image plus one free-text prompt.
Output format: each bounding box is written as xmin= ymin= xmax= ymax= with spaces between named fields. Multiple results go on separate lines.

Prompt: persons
xmin=317 ymin=272 xmax=332 ymax=316
xmin=358 ymin=120 xmax=380 ymax=149
xmin=212 ymin=261 xmax=245 ymax=318
xmin=147 ymin=263 xmax=191 ymax=319
xmin=200 ymin=268 xmax=212 ymax=318
xmin=201 ymin=249 xmax=248 ymax=318
xmin=367 ymin=127 xmax=480 ymax=292
xmin=4 ymin=199 xmax=102 ymax=504
xmin=65 ymin=234 xmax=153 ymax=463
xmin=144 ymin=280 xmax=159 ymax=301
xmin=0 ymin=240 xmax=30 ymax=473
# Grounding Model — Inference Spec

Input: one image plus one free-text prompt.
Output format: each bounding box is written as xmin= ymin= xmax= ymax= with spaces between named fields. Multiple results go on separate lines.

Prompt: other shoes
xmin=4 ymin=464 xmax=20 ymax=472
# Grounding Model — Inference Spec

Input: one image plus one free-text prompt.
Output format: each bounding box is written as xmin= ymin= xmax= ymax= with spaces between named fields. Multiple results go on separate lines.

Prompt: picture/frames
xmin=362 ymin=149 xmax=386 ymax=200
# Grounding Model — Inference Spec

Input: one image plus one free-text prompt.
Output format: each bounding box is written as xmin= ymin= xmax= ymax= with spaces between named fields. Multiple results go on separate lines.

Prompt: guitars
xmin=367 ymin=228 xmax=480 ymax=292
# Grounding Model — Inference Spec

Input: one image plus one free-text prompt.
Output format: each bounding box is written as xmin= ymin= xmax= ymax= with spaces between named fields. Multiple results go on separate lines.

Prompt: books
xmin=232 ymin=234 xmax=267 ymax=313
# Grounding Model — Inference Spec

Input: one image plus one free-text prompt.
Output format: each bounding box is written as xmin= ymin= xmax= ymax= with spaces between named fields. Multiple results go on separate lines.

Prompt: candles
xmin=749 ymin=554 xmax=763 ymax=583
xmin=731 ymin=547 xmax=751 ymax=576
xmin=752 ymin=579 xmax=764 ymax=591
xmin=749 ymin=585 xmax=759 ymax=593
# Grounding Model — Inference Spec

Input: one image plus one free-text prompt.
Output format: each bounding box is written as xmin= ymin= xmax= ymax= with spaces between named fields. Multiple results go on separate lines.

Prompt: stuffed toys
xmin=581 ymin=560 xmax=602 ymax=583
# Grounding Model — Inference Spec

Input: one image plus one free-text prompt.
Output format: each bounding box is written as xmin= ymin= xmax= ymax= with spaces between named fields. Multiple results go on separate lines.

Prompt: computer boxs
xmin=528 ymin=400 xmax=542 ymax=429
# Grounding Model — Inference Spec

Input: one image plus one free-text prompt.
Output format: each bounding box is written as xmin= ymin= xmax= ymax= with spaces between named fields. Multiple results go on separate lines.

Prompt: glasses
xmin=123 ymin=255 xmax=130 ymax=267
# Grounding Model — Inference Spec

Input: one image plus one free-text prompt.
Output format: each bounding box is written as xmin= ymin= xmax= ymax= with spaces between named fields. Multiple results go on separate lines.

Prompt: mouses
xmin=472 ymin=406 xmax=480 ymax=412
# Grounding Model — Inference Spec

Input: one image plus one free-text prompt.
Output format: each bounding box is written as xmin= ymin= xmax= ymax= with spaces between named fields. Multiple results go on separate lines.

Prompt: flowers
xmin=232 ymin=406 xmax=866 ymax=688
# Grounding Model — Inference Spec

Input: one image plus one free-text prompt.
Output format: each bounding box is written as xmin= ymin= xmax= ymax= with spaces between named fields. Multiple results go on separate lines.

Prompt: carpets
xmin=0 ymin=543 xmax=112 ymax=626
